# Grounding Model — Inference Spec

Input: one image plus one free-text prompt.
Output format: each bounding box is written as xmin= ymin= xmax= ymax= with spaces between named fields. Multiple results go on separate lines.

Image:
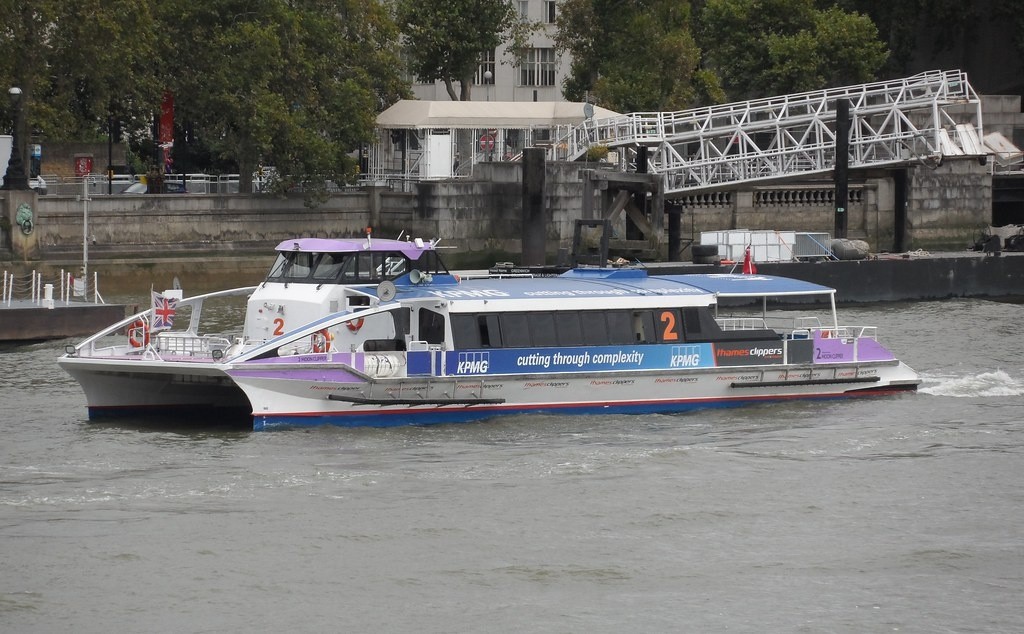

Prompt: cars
xmin=114 ymin=182 xmax=189 ymax=194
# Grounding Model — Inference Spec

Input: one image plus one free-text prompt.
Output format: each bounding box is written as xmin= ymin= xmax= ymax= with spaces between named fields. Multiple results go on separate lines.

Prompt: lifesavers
xmin=311 ymin=329 xmax=330 ymax=353
xmin=127 ymin=321 xmax=150 ymax=347
xmin=347 ymin=317 xmax=364 ymax=331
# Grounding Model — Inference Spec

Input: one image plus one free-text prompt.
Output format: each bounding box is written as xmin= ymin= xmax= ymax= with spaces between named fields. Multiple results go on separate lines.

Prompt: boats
xmin=58 ymin=229 xmax=924 ymax=433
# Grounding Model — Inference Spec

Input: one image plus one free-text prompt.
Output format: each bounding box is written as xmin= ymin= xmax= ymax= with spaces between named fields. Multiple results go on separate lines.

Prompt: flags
xmin=153 ymin=297 xmax=177 ymax=326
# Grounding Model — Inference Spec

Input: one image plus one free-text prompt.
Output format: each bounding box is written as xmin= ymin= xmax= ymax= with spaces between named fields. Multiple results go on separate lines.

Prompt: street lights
xmin=483 ymin=70 xmax=492 ymax=162
